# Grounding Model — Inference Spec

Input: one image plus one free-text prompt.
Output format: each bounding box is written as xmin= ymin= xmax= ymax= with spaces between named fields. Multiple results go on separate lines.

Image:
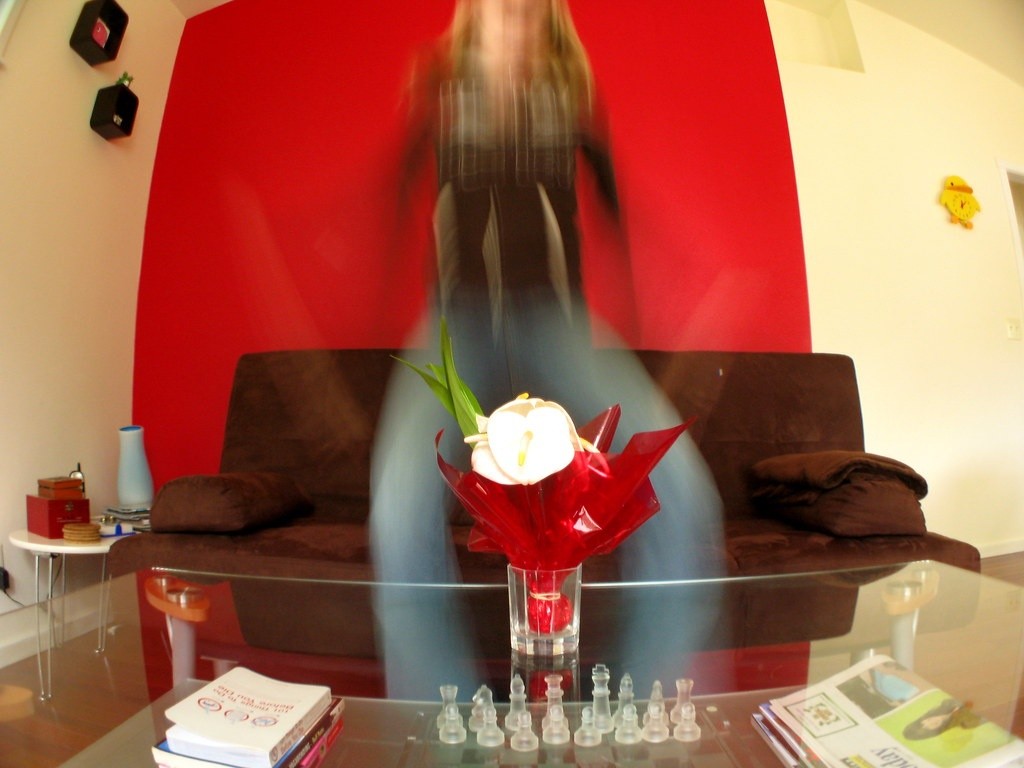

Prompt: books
xmin=151 ymin=667 xmax=345 ymax=768
xmin=750 ymin=653 xmax=1024 ymax=768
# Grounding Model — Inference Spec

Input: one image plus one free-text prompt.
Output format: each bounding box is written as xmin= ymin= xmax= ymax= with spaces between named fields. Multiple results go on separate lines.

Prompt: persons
xmin=902 ymin=698 xmax=981 ymax=740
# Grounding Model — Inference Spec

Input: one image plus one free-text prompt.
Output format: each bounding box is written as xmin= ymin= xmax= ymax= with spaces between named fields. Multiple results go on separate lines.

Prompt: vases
xmin=506 ymin=564 xmax=583 ymax=658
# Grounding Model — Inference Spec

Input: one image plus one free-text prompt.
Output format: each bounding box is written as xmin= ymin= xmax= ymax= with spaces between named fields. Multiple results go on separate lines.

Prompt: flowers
xmin=392 ymin=316 xmax=700 ymax=630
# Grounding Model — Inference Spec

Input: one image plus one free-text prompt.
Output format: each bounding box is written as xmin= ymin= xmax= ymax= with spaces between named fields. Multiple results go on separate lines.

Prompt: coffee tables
xmin=0 ymin=557 xmax=1024 ymax=768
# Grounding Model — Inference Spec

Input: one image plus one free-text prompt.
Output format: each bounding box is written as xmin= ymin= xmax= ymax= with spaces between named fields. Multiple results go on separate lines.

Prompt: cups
xmin=506 ymin=562 xmax=583 ymax=657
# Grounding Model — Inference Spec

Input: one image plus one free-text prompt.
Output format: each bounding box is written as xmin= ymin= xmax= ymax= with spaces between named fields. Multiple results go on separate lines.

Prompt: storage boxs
xmin=27 ymin=494 xmax=90 ymax=539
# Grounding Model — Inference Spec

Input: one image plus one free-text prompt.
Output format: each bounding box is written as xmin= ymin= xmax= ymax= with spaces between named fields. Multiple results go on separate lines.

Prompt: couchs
xmin=150 ymin=347 xmax=981 ymax=660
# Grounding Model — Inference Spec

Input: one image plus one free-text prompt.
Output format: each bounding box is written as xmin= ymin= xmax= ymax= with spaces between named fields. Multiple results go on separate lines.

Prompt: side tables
xmin=10 ymin=518 xmax=149 ymax=700
